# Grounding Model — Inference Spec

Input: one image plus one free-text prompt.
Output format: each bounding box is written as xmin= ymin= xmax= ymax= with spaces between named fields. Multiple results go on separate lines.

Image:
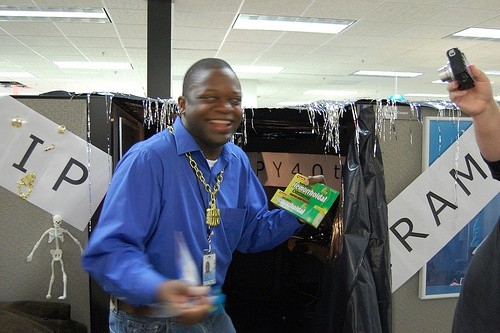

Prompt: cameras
xmin=437 ymin=47 xmax=477 ymax=92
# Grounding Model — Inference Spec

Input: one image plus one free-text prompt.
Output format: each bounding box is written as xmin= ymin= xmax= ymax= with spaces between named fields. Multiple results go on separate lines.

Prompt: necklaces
xmin=166 ymin=124 xmax=225 ymax=226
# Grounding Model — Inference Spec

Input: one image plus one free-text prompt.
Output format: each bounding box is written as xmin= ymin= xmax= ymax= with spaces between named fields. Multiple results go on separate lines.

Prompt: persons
xmin=203 ymin=262 xmax=215 ymax=282
xmin=80 ymin=57 xmax=326 ymax=333
xmin=452 ymin=66 xmax=500 ymax=333
